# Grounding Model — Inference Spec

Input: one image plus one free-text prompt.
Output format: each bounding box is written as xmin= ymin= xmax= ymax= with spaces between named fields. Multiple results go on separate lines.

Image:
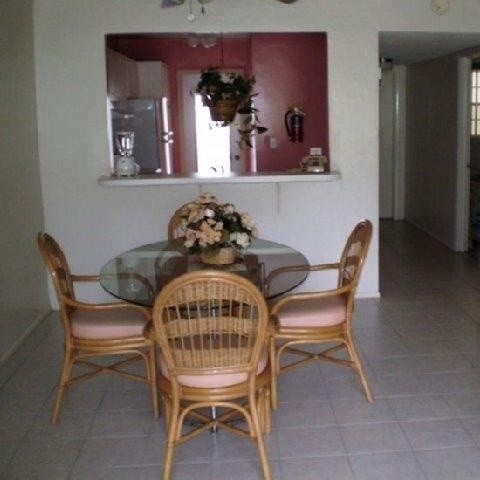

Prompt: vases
xmin=201 ymin=247 xmax=237 ymax=264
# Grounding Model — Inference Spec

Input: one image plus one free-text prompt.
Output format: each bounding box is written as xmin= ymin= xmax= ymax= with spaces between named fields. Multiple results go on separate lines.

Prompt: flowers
xmin=178 ymin=193 xmax=259 ymax=254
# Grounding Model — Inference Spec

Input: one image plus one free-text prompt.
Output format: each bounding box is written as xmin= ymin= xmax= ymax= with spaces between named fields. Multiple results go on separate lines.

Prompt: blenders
xmin=116 ymin=130 xmax=140 ymax=178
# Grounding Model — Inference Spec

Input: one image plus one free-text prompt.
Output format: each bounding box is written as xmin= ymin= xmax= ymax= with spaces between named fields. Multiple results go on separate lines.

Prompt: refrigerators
xmin=128 ymin=98 xmax=172 ymax=174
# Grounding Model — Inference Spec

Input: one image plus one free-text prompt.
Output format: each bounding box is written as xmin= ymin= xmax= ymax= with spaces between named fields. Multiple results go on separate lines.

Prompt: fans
xmin=162 ymin=0 xmax=298 ymax=22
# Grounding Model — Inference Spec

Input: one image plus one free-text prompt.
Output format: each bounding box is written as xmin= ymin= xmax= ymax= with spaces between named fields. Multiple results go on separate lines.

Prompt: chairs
xmin=265 ymin=219 xmax=375 ymax=409
xmin=167 ymin=211 xmax=195 ymax=239
xmin=37 ymin=231 xmax=159 ymax=427
xmin=141 ymin=270 xmax=276 ymax=480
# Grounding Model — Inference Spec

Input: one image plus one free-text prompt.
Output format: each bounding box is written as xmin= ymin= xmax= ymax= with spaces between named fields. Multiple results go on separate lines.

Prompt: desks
xmin=99 ymin=237 xmax=310 ymax=432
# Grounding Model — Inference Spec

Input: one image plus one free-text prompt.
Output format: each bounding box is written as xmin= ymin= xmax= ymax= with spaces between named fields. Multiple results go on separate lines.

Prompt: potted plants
xmin=189 ymin=64 xmax=268 ymax=161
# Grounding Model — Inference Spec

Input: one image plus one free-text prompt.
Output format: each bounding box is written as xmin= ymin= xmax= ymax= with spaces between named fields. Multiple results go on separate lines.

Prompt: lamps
xmin=187 ymin=33 xmax=217 ymax=48
xmin=432 ymin=0 xmax=451 ymax=14
